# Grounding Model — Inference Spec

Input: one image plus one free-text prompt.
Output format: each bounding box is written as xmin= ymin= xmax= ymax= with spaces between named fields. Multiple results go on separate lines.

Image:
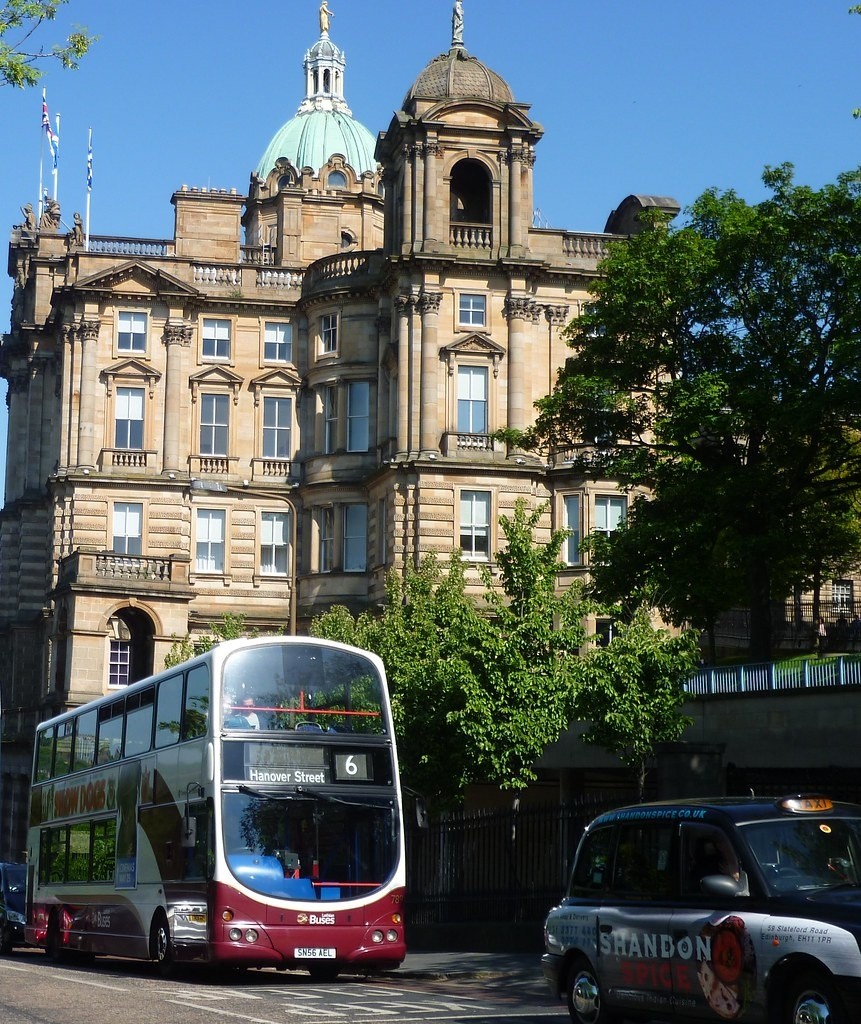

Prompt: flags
xmin=42 ymin=97 xmax=52 ymax=148
xmin=52 ymin=122 xmax=59 ymax=175
xmin=86 ymin=130 xmax=93 ymax=193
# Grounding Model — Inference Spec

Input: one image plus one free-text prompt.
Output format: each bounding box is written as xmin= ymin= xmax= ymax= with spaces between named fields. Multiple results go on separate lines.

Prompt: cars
xmin=0 ymin=862 xmax=32 ymax=957
xmin=540 ymin=794 xmax=861 ymax=1024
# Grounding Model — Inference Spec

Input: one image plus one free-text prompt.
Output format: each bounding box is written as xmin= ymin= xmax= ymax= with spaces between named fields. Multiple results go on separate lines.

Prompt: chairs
xmin=295 ymin=720 xmax=338 ymax=733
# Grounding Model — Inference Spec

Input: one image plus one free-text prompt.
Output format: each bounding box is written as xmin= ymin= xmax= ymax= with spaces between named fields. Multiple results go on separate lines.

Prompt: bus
xmin=24 ymin=635 xmax=432 ymax=983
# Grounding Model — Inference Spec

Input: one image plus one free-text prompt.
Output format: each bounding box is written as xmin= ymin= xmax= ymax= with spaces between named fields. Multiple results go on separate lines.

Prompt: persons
xmin=704 ymin=829 xmax=749 ymax=885
xmin=74 ymin=211 xmax=84 ymax=244
xmin=40 ymin=196 xmax=62 ymax=229
xmin=296 ymin=818 xmax=314 ymax=866
xmin=452 ymin=1 xmax=464 ymax=43
xmin=727 ymin=761 xmax=741 ymax=784
xmin=20 ymin=203 xmax=37 ymax=230
xmin=234 ymin=694 xmax=261 ymax=732
xmin=319 ymin=1 xmax=335 ymax=31
